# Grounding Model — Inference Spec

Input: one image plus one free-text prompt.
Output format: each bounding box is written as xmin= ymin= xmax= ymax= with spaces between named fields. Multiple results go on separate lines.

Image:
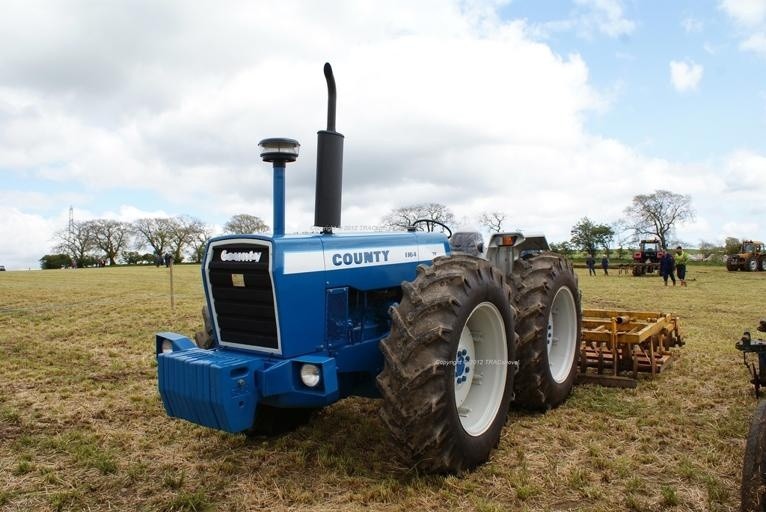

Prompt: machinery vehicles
xmin=157 ymin=62 xmax=686 ymax=476
xmin=619 ymin=240 xmax=664 ymax=274
xmin=726 ymin=240 xmax=766 ymax=271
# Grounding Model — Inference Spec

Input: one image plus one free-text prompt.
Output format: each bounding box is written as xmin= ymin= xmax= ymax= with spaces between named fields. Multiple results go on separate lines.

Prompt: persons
xmin=70 ymin=258 xmax=107 ymax=269
xmin=600 ymin=255 xmax=609 ymax=275
xmin=586 ymin=254 xmax=597 ymax=276
xmin=657 ymin=245 xmax=690 ymax=288
xmin=153 ymin=253 xmax=172 ymax=269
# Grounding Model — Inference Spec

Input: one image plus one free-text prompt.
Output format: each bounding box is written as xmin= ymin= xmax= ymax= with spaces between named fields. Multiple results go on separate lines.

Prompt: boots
xmin=664 ymin=279 xmax=686 ymax=287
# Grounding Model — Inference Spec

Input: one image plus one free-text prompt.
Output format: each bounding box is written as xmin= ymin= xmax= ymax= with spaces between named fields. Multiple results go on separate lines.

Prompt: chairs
xmin=450 ymin=231 xmax=484 ymax=256
